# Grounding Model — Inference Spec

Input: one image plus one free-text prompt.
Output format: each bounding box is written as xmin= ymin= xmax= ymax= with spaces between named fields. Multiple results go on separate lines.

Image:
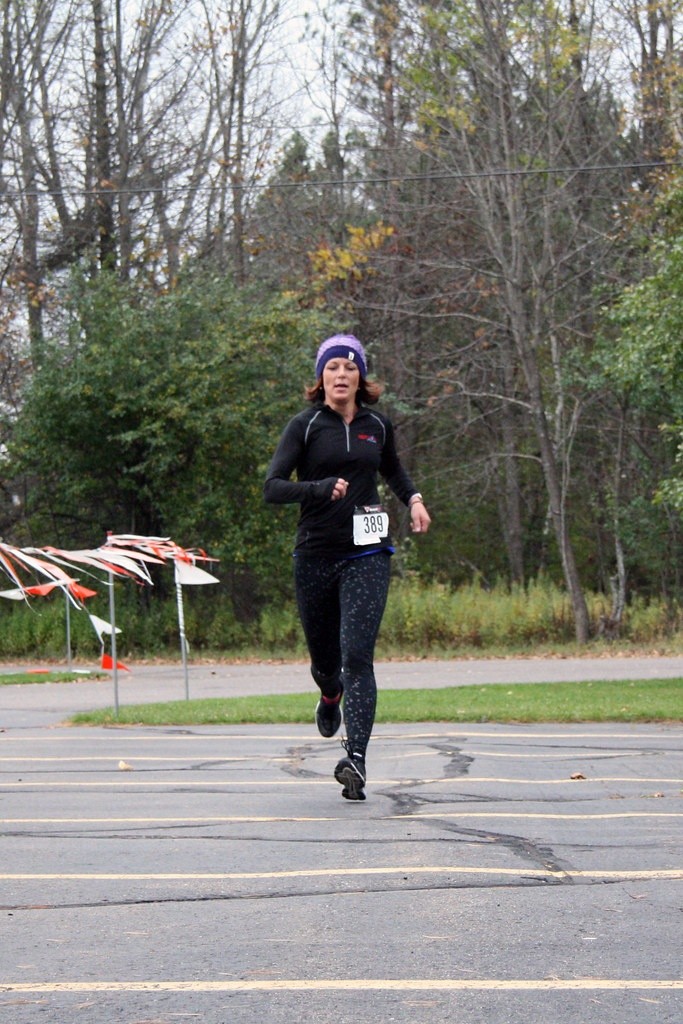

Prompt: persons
xmin=263 ymin=333 xmax=432 ymax=800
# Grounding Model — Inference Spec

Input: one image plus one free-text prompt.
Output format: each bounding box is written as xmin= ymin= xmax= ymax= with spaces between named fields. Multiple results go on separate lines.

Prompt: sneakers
xmin=334 ymin=737 xmax=367 ymax=800
xmin=315 ymin=674 xmax=345 ymax=738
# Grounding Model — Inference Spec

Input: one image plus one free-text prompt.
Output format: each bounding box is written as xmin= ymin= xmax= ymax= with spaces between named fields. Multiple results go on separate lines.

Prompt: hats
xmin=316 ymin=334 xmax=366 ymax=379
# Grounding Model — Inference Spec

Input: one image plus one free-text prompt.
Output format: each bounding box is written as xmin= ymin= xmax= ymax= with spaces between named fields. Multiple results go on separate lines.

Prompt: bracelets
xmin=408 ymin=493 xmax=423 ymax=508
